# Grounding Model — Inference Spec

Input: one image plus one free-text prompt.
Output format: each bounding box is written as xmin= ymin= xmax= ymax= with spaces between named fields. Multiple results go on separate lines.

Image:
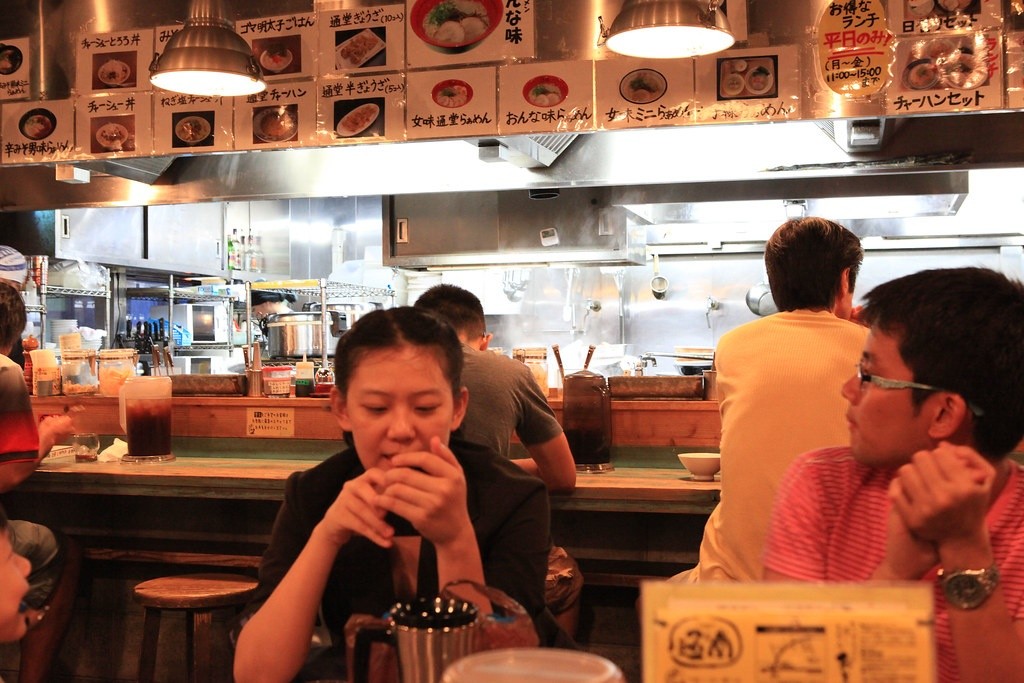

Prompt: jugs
xmin=119 ymin=376 xmax=176 ymax=466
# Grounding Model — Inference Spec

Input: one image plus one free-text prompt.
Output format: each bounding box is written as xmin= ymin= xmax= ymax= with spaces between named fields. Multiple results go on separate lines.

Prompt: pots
xmin=266 ymin=311 xmax=347 ymax=360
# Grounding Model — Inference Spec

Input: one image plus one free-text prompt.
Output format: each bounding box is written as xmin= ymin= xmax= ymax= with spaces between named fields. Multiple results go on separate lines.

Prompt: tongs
xmin=151 ymin=344 xmax=162 ymax=376
xmin=163 ymin=346 xmax=174 ymax=375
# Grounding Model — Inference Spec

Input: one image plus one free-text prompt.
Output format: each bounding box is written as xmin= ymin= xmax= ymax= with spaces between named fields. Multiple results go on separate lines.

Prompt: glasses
xmin=856 ymin=364 xmax=985 ymax=415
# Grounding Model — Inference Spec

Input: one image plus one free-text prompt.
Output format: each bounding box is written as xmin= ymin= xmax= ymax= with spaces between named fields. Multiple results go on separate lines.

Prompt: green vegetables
xmin=532 ymin=85 xmax=544 ymax=95
xmin=752 ymin=66 xmax=770 ymax=77
xmin=8 ymin=51 xmax=20 ymax=61
xmin=429 ymin=2 xmax=455 ymax=25
xmin=267 ymin=42 xmax=287 ymax=57
xmin=631 ymin=77 xmax=646 ymax=89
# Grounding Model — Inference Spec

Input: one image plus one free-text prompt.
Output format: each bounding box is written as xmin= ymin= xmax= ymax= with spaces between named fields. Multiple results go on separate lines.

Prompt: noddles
xmin=422 ymin=0 xmax=491 ymax=45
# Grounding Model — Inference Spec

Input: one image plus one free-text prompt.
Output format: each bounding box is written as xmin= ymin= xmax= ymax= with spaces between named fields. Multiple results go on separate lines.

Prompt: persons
xmin=635 ymin=217 xmax=871 ymax=615
xmin=0 ymin=245 xmax=81 ymax=682
xmin=761 ymin=266 xmax=1024 ymax=683
xmin=413 ymin=283 xmax=582 ymax=642
xmin=233 ymin=306 xmax=583 ymax=682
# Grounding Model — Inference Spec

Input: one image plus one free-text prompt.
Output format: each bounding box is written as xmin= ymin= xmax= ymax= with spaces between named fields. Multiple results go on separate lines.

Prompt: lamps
xmin=149 ymin=0 xmax=268 ymax=96
xmin=596 ymin=0 xmax=736 ymax=58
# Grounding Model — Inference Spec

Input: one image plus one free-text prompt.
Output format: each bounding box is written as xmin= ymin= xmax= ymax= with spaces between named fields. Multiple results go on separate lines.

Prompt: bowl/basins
xmin=0 ymin=43 xmax=294 ymax=148
xmin=410 ymin=0 xmax=773 ymax=111
xmin=45 ymin=343 xmax=56 ymax=349
xmin=900 ymin=0 xmax=989 ymax=90
xmin=677 ymin=453 xmax=720 ymax=481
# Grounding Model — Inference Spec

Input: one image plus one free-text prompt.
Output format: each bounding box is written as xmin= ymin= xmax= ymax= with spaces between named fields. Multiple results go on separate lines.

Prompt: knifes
xmin=126 ymin=317 xmax=165 ymax=339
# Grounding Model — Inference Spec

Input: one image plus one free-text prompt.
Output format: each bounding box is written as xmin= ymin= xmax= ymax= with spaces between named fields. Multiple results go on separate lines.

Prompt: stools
xmin=136 ymin=573 xmax=259 ymax=682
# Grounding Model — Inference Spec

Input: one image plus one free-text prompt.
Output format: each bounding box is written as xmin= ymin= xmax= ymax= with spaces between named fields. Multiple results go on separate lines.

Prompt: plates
xmin=250 ymin=26 xmax=385 ymax=140
xmin=50 ymin=319 xmax=102 ymax=351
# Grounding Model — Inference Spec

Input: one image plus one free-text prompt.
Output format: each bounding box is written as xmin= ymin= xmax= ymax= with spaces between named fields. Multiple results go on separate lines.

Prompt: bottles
xmin=228 ymin=228 xmax=257 ymax=273
xmin=99 ymin=348 xmax=138 ymax=397
xmin=61 ymin=347 xmax=99 ymax=396
xmin=295 ymin=352 xmax=315 ymax=398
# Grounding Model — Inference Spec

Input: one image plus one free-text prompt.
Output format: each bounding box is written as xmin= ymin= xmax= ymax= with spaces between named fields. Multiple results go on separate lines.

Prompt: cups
xmin=344 ymin=591 xmax=479 ymax=683
xmin=32 ymin=367 xmax=60 ymax=395
xmin=439 ymin=646 xmax=626 ymax=683
xmin=73 ymin=433 xmax=100 ymax=463
xmin=562 ymin=369 xmax=615 ymax=474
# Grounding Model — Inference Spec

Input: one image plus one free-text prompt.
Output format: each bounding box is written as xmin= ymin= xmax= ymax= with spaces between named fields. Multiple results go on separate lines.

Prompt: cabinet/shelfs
xmin=0 ymin=202 xmax=230 ymax=283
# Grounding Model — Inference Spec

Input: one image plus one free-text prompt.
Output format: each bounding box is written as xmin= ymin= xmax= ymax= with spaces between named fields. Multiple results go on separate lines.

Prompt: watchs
xmin=938 ymin=558 xmax=998 ymax=608
